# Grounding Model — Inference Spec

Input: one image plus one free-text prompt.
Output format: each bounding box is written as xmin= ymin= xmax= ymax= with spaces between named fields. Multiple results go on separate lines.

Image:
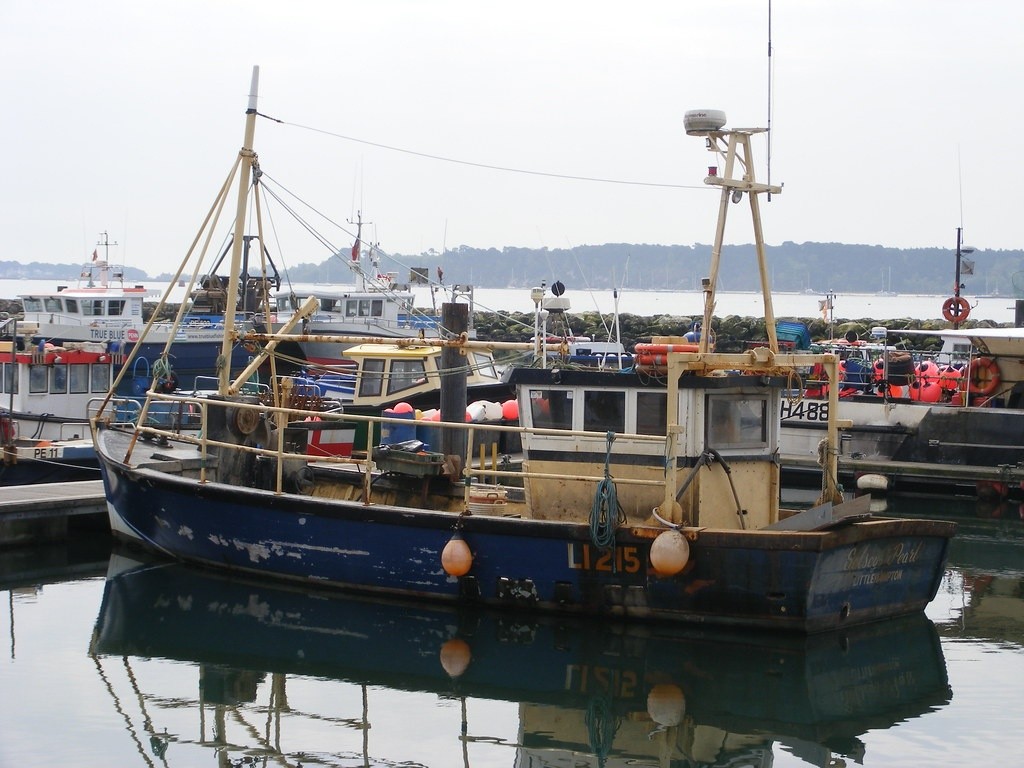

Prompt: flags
xmin=352 ymin=235 xmax=359 ymax=261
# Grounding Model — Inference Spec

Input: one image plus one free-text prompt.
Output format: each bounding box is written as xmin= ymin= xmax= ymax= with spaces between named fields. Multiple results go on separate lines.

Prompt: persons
xmin=438 ymin=266 xmax=443 ymax=284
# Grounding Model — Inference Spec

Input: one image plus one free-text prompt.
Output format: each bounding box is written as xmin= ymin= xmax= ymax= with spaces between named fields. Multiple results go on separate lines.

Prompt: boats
xmin=0 ymin=0 xmax=1023 ymax=637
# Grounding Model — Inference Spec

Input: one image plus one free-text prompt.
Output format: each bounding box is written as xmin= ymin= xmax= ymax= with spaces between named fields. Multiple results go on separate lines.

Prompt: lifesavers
xmin=160 ymin=371 xmax=178 ymax=393
xmin=963 ymin=358 xmax=999 ymax=395
xmin=630 ymin=341 xmax=702 ymax=365
xmin=942 ymin=296 xmax=969 ymax=323
